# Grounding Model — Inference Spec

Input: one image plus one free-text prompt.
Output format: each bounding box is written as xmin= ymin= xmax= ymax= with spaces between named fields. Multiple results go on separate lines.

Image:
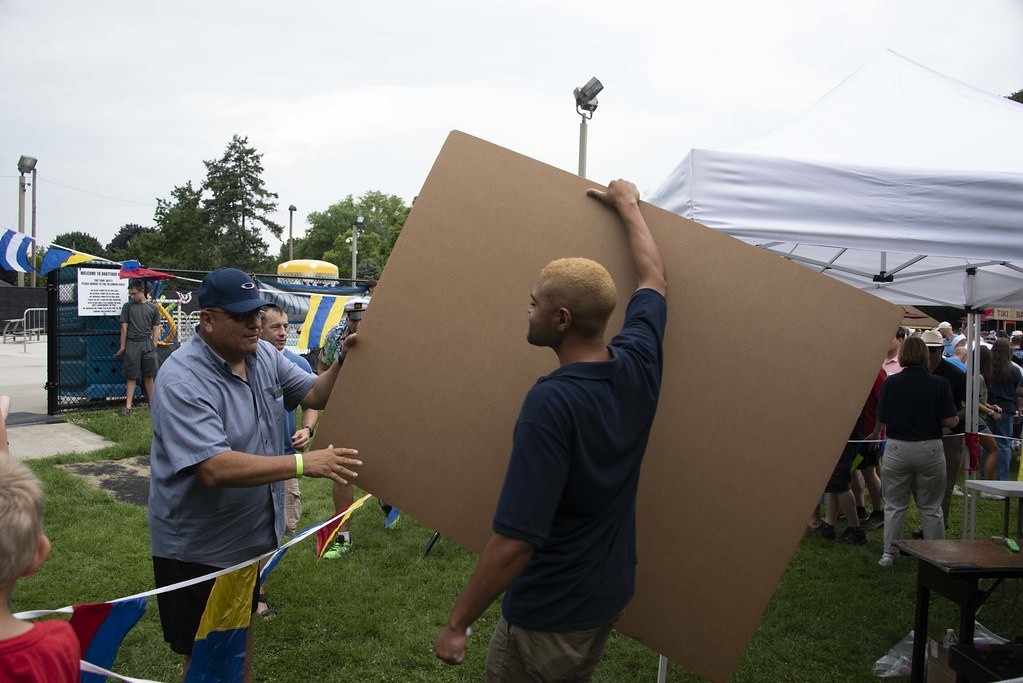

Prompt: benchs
xmin=3 ymin=319 xmax=44 ymax=345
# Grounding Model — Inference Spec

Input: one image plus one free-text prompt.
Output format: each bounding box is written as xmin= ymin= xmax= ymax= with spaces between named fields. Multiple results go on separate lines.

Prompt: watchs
xmin=303 ymin=424 xmax=313 ymax=438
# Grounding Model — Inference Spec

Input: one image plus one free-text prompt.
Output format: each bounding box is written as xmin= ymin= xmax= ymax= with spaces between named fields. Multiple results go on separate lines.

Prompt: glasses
xmin=206 ymin=307 xmax=267 ymax=322
xmin=986 ymin=338 xmax=996 ymax=340
xmin=927 ymin=347 xmax=942 ymax=353
xmin=129 ymin=289 xmax=143 ymax=296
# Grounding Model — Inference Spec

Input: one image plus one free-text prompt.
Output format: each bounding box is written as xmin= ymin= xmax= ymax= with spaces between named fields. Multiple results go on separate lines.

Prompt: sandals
xmin=259 ymin=594 xmax=276 ymax=621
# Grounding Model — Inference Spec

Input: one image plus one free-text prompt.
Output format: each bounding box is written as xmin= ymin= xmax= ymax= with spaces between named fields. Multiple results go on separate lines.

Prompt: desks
xmin=889 ymin=538 xmax=1022 ymax=682
xmin=965 ymin=479 xmax=1023 ymax=600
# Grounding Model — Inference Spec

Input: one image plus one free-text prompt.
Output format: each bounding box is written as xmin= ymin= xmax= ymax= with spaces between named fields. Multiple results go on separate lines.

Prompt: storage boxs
xmin=927 ymin=620 xmax=1007 ymax=682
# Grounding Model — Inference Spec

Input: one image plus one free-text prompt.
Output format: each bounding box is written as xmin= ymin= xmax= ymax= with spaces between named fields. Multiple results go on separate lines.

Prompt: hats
xmin=983 ymin=334 xmax=997 ymax=344
xmin=1010 ymin=330 xmax=1023 ymax=339
xmin=907 ymin=328 xmax=915 ymax=337
xmin=198 ymin=268 xmax=278 ymax=313
xmin=995 ymin=330 xmax=1007 ymax=337
xmin=936 ymin=321 xmax=952 ymax=331
xmin=989 ymin=330 xmax=997 ymax=335
xmin=343 ymin=298 xmax=370 ymax=321
xmin=919 ymin=330 xmax=952 ymax=346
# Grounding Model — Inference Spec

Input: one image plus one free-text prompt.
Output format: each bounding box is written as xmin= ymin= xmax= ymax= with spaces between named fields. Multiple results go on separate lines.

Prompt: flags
xmin=317 ymin=498 xmax=364 ymax=563
xmin=68 ymin=596 xmax=149 ymax=683
xmin=181 ymin=521 xmax=323 ymax=683
xmin=119 ymin=260 xmax=178 ymax=282
xmin=0 ymin=229 xmax=37 ymax=274
xmin=39 ymin=244 xmax=100 ymax=279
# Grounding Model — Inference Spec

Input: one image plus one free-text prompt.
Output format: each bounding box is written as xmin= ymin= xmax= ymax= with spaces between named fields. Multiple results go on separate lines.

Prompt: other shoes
xmin=980 ymin=491 xmax=1005 ymax=500
xmin=813 ymin=520 xmax=836 ymax=541
xmin=952 ymin=484 xmax=971 ymax=497
xmin=879 ymin=555 xmax=895 ymax=567
xmin=839 ymin=526 xmax=868 ymax=547
xmin=866 ymin=509 xmax=884 ymax=528
xmin=120 ymin=406 xmax=132 ymax=416
xmin=857 ymin=509 xmax=869 ymax=521
xmin=807 ymin=519 xmax=823 ymax=529
xmin=913 ymin=522 xmax=949 ymax=538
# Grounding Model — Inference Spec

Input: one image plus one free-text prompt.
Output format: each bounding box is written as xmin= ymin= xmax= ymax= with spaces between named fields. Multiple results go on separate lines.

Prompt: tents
xmin=644 ymin=147 xmax=1023 ymax=544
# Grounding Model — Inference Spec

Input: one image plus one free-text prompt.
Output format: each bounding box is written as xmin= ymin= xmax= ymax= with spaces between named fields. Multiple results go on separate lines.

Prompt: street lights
xmin=573 ymin=76 xmax=603 ymax=178
xmin=17 ymin=154 xmax=39 ymax=288
xmin=352 ymin=214 xmax=364 ymax=287
xmin=288 ymin=204 xmax=298 ymax=261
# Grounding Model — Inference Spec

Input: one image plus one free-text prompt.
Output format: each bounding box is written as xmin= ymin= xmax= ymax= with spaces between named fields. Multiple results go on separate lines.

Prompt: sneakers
xmin=380 ymin=500 xmax=401 ymax=530
xmin=322 ymin=535 xmax=353 ymax=559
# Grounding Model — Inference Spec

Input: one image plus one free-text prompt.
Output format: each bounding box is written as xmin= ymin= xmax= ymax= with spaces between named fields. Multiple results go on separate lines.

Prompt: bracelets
xmin=989 ymin=410 xmax=994 ymax=416
xmin=294 ymin=453 xmax=303 ymax=478
xmin=338 ymin=353 xmax=344 ymax=364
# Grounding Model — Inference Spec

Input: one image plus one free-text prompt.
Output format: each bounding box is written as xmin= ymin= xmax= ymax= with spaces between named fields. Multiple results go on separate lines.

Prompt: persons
xmin=432 ymin=178 xmax=667 ymax=683
xmin=317 ymin=296 xmax=369 ymax=375
xmin=364 ymin=280 xmax=378 ymax=301
xmin=147 ymin=263 xmax=363 ymax=683
xmin=113 ymin=280 xmax=161 ymax=416
xmin=803 ymin=321 xmax=1023 ymax=567
xmin=0 ymin=453 xmax=81 ymax=683
xmin=259 ymin=300 xmax=319 ymax=456
